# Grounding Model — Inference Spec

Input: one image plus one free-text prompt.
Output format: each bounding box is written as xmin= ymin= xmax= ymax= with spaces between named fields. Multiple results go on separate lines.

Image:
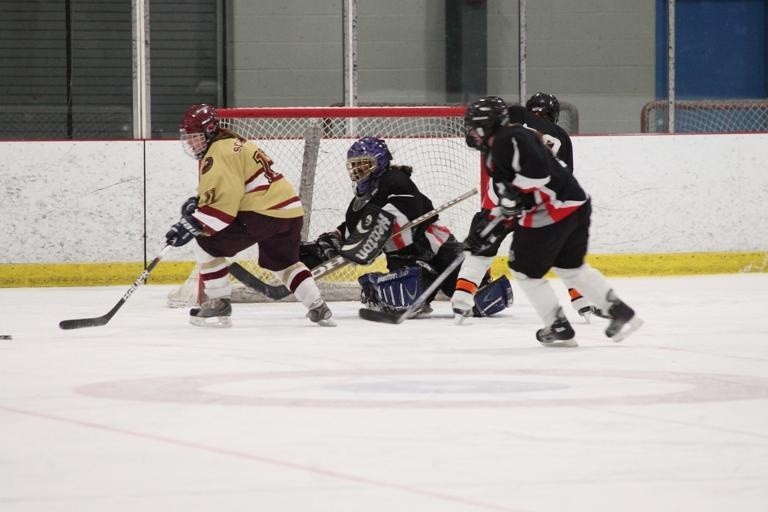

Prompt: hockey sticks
xmin=359 ymin=215 xmax=506 ymax=324
xmin=230 ymin=187 xmax=477 ymax=299
xmin=59 ymin=205 xmax=196 ymax=328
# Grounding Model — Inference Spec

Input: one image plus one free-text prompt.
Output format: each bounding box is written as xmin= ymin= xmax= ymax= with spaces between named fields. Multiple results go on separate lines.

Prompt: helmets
xmin=464 ymin=96 xmax=509 ymax=151
xmin=346 ymin=137 xmax=393 ymax=196
xmin=527 ymin=92 xmax=560 ymax=125
xmin=179 ymin=103 xmax=220 ymax=160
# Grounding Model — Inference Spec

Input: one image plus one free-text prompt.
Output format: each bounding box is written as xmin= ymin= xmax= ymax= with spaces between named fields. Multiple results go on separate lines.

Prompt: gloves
xmin=166 ymin=216 xmax=203 ymax=246
xmin=182 ymin=196 xmax=199 ymax=215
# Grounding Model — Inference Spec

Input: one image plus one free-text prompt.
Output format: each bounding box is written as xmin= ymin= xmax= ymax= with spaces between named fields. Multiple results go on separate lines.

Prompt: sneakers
xmin=536 ymin=318 xmax=574 ymax=342
xmin=606 ymin=302 xmax=634 ymax=337
xmin=308 ymin=297 xmax=331 ymax=322
xmin=451 ymin=289 xmax=475 ymax=317
xmin=571 ymin=297 xmax=596 ymax=313
xmin=190 ymin=298 xmax=231 ymax=317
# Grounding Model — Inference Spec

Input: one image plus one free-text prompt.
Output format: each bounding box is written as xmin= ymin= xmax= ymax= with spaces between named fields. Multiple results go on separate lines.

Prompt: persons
xmin=524 ymin=92 xmax=591 ymax=312
xmin=315 ymin=136 xmax=491 ymax=317
xmin=463 ymin=96 xmax=636 ymax=344
xmin=166 ymin=105 xmax=331 ymax=322
